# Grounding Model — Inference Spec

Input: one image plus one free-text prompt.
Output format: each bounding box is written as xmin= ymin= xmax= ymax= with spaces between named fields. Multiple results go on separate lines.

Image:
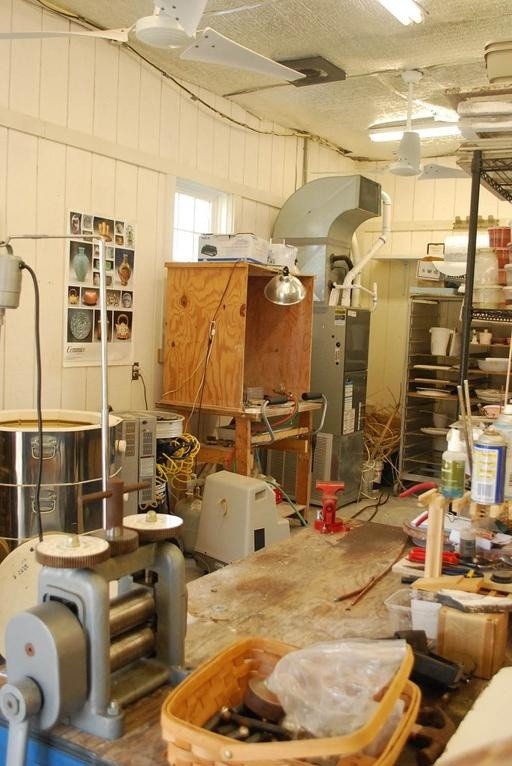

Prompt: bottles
xmin=439 ymin=428 xmax=467 ymax=500
xmin=470 ymin=403 xmax=512 ymax=506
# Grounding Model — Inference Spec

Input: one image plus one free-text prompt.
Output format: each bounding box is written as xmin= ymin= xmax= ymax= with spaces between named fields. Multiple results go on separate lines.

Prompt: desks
xmin=52 ymin=517 xmax=493 ymax=766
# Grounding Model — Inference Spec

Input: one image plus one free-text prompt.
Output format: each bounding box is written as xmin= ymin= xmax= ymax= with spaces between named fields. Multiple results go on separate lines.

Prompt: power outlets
xmin=132 ymin=361 xmax=140 ymax=380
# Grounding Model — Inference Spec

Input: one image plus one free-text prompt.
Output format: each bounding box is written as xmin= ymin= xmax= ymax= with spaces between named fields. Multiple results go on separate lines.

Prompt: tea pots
xmin=114 ymin=314 xmax=130 ymax=339
xmin=68 ymin=289 xmax=79 ymax=304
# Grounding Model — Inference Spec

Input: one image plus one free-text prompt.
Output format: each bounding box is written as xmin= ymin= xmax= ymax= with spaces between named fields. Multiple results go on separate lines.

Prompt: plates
xmin=70 ymin=312 xmax=91 ymax=340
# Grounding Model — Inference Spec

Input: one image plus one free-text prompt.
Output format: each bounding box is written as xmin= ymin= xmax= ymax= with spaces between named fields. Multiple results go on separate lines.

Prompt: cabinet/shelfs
xmin=393 ymin=294 xmax=466 ymax=497
xmin=453 ymin=149 xmax=512 ymax=422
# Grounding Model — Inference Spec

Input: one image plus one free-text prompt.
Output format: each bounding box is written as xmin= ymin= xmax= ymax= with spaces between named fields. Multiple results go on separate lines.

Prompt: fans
xmin=305 ymin=69 xmax=472 ymax=181
xmin=0 ymin=0 xmax=308 ymax=83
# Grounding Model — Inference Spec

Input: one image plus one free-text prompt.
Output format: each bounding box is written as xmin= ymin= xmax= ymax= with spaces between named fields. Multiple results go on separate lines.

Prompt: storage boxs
xmin=384 ymin=588 xmax=510 ymax=680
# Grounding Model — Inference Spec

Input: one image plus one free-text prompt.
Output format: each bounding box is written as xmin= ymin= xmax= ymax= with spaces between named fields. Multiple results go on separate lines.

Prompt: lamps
xmin=376 ymin=1 xmax=430 ymax=27
xmin=263 ymin=266 xmax=308 ymax=306
xmin=367 ymin=122 xmax=462 ymax=143
